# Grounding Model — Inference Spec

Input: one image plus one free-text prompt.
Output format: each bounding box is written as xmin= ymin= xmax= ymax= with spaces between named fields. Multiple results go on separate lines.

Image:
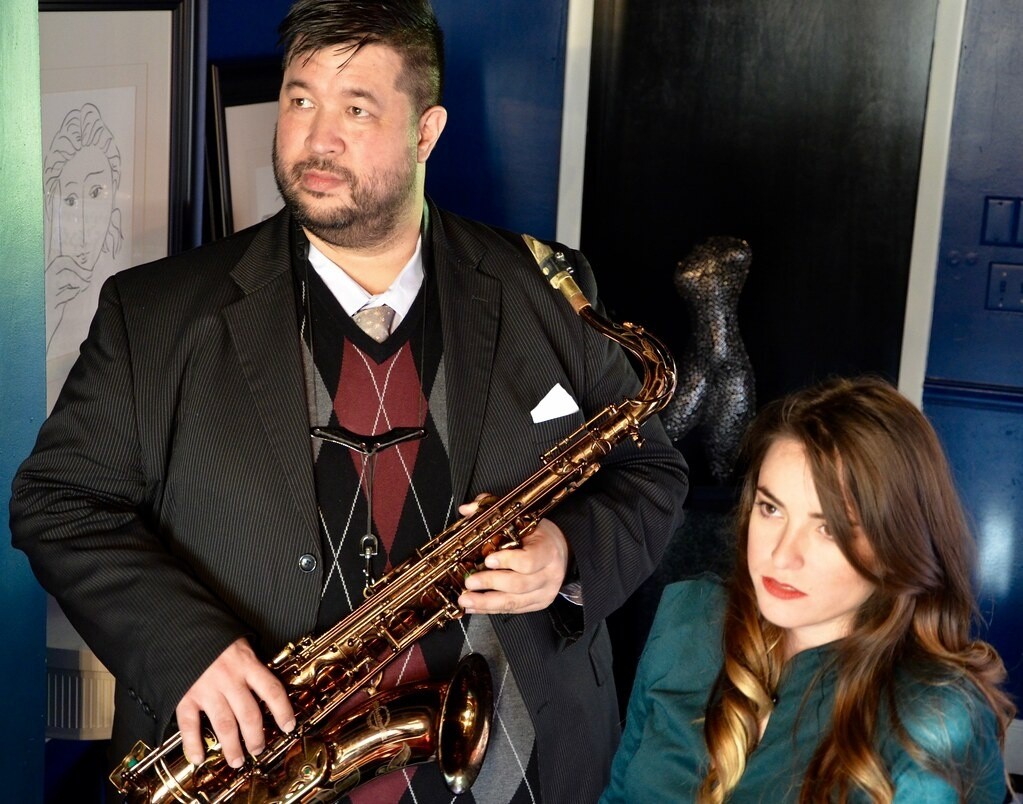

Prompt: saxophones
xmin=106 ymin=232 xmax=680 ymax=804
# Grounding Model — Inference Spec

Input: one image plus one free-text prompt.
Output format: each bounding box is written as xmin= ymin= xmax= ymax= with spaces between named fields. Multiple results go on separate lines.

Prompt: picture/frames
xmin=39 ymin=0 xmax=196 ymax=417
xmin=209 ymin=63 xmax=286 ymax=238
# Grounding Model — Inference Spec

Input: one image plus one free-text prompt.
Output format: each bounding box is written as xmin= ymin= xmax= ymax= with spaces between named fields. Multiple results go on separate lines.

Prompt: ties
xmin=350 ymin=304 xmax=395 ymax=344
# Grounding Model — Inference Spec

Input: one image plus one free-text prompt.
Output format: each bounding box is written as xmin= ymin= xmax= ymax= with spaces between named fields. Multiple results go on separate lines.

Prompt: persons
xmin=600 ymin=375 xmax=1022 ymax=804
xmin=8 ymin=1 xmax=691 ymax=804
